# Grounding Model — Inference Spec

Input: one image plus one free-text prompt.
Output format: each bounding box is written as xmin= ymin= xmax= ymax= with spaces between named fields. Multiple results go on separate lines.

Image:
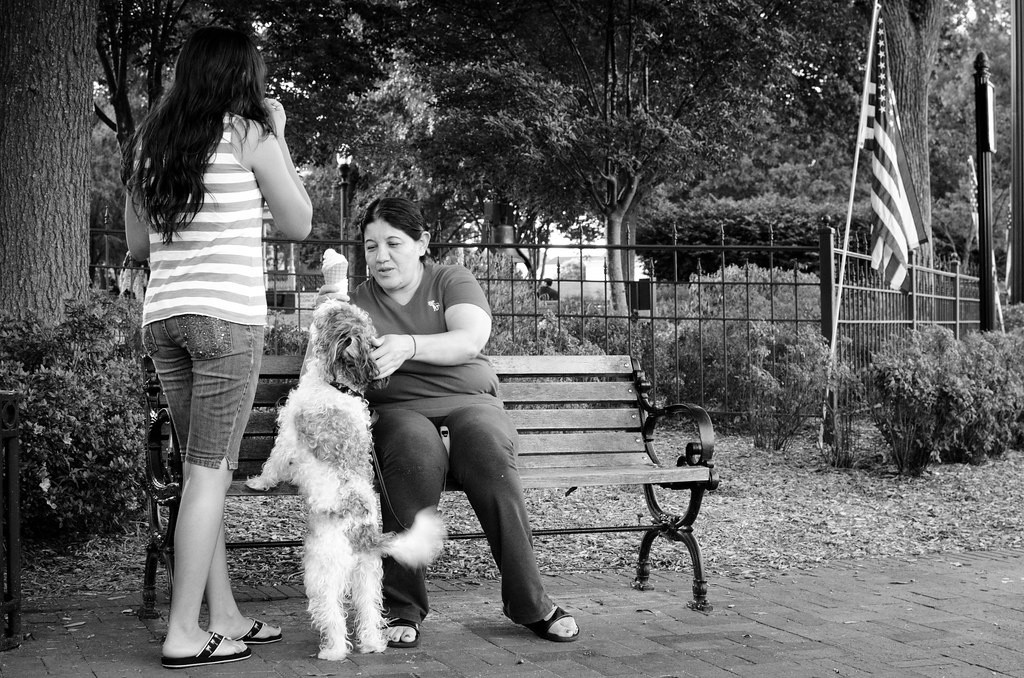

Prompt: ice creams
xmin=322 ymin=248 xmax=349 ymax=296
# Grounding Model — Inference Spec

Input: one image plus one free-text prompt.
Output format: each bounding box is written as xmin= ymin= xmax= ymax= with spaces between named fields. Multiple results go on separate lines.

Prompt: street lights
xmin=336 ymin=143 xmax=353 ymax=257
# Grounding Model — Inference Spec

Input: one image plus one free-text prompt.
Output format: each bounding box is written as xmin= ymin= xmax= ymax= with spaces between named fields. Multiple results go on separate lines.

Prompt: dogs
xmin=245 ymin=298 xmax=448 ymax=662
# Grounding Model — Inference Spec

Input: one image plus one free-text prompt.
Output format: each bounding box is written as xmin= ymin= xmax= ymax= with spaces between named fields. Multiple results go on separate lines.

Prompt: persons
xmin=123 ymin=25 xmax=316 ymax=667
xmin=310 ymin=196 xmax=578 ymax=642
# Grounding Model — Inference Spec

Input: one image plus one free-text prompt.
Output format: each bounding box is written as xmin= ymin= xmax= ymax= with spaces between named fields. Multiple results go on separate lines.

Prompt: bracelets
xmin=407 ymin=334 xmax=417 ymax=361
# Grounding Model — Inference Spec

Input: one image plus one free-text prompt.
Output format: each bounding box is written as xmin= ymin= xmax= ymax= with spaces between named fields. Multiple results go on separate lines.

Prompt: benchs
xmin=135 ymin=353 xmax=722 ymax=613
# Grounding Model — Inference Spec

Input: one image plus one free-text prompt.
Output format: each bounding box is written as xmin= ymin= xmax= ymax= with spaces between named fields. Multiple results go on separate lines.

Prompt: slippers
xmin=524 ymin=604 xmax=578 ymax=642
xmin=378 ymin=618 xmax=420 ymax=648
xmin=234 ymin=618 xmax=284 ymax=645
xmin=161 ymin=631 xmax=252 ymax=669
